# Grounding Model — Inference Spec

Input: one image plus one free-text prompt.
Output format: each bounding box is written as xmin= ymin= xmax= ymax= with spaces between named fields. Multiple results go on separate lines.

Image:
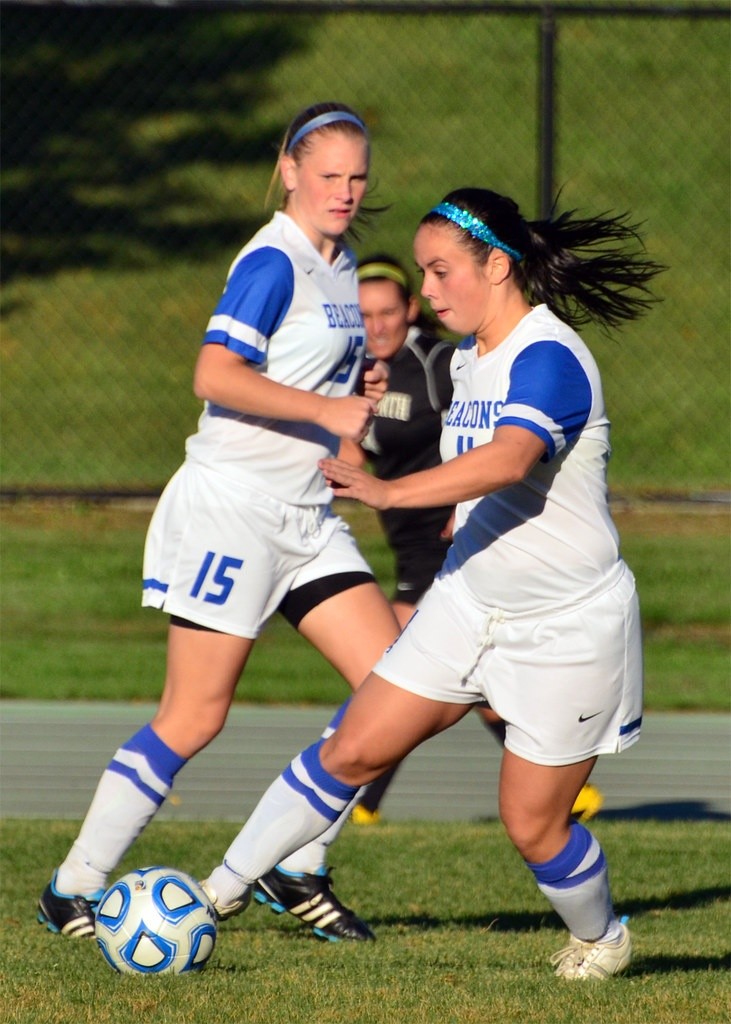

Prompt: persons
xmin=36 ymin=102 xmax=402 ymax=943
xmin=347 ymin=251 xmax=604 ymax=828
xmin=197 ymin=186 xmax=669 ymax=981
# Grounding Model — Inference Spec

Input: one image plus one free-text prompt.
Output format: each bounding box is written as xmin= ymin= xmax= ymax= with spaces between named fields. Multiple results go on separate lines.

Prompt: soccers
xmin=94 ymin=867 xmax=217 ymax=977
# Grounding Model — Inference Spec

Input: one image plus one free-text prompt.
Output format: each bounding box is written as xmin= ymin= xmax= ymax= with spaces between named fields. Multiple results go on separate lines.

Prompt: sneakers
xmin=549 ymin=915 xmax=632 ymax=981
xmin=252 ymin=863 xmax=378 ymax=945
xmin=36 ymin=868 xmax=107 ymax=940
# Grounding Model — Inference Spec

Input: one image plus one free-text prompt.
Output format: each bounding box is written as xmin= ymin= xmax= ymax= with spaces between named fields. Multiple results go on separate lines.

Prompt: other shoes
xmin=569 ymin=782 xmax=605 ymax=822
xmin=351 ymin=803 xmax=381 ymax=825
